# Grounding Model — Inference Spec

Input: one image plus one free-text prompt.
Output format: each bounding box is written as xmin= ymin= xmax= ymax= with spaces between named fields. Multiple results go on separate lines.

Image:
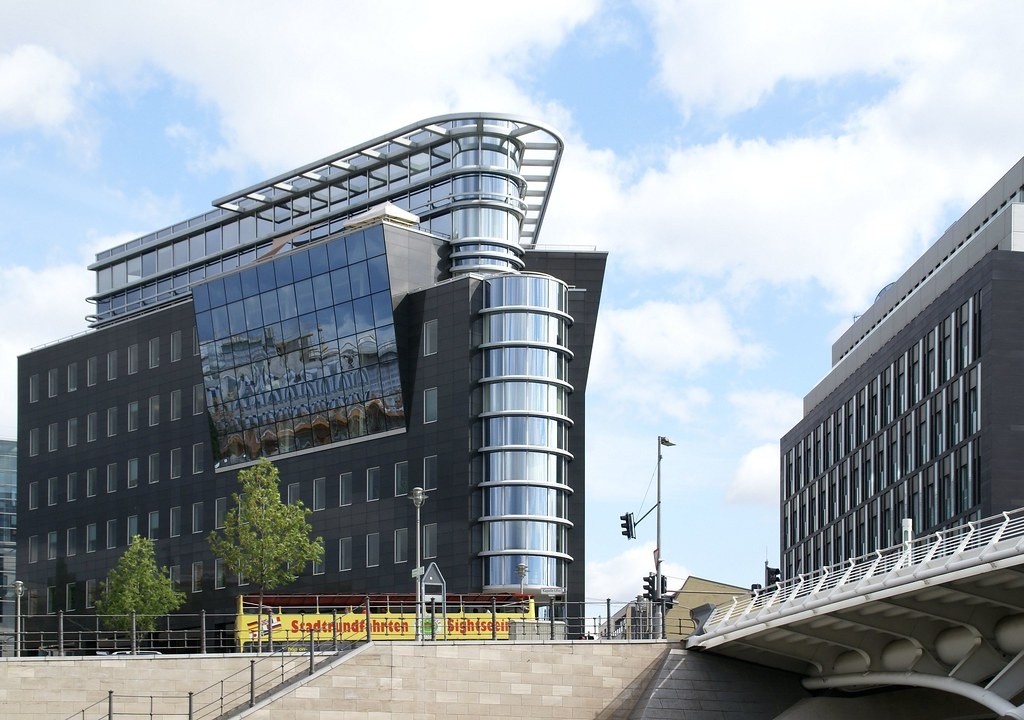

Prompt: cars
xmin=109 ymin=651 xmax=162 ymax=655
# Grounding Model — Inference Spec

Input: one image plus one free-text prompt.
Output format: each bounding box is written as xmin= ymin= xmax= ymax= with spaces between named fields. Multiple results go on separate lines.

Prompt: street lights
xmin=14 ymin=580 xmax=24 ymax=652
xmin=655 ymin=435 xmax=677 ymax=640
xmin=411 ymin=487 xmax=425 ymax=642
xmin=515 ymin=562 xmax=528 ymax=633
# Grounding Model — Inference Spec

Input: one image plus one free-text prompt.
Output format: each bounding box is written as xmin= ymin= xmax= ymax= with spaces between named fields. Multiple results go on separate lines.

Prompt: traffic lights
xmin=751 ymin=584 xmax=762 ymax=607
xmin=642 ymin=576 xmax=652 ymax=601
xmin=620 ymin=514 xmax=629 ymax=538
xmin=768 ymin=568 xmax=780 ymax=597
xmin=662 ymin=576 xmax=668 ymax=594
